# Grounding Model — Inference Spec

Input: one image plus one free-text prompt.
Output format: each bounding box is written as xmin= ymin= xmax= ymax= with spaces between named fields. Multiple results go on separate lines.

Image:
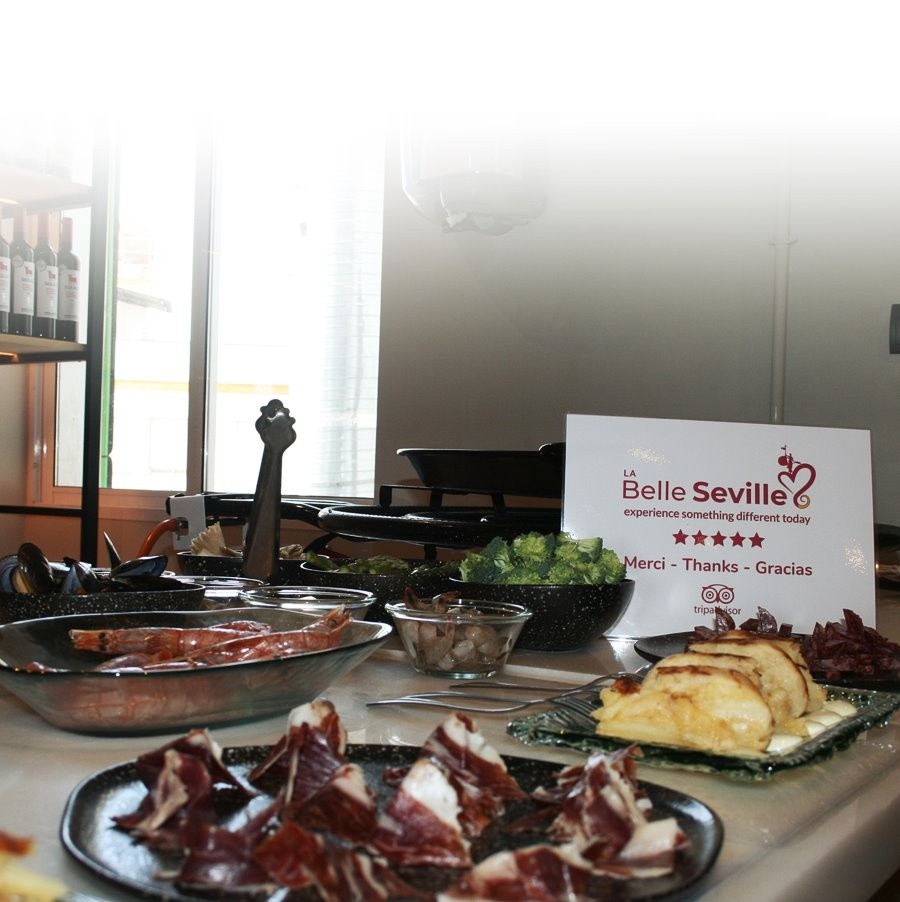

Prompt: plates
xmin=633 ymin=631 xmax=900 ymax=685
xmin=505 ymin=685 xmax=899 ymax=784
xmin=58 ymin=744 xmax=722 ymax=902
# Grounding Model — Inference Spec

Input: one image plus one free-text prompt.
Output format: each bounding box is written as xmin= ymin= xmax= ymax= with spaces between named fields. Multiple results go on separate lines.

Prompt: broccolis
xmin=458 ymin=529 xmax=627 ymax=585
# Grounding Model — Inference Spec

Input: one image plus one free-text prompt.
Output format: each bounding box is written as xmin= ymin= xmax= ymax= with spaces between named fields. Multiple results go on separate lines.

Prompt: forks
xmin=366 ymin=695 xmax=601 ymax=725
xmin=449 ymin=670 xmax=644 ymax=692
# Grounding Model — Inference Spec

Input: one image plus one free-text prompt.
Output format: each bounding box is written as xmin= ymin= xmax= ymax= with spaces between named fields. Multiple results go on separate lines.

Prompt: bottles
xmin=0 ymin=203 xmax=80 ymax=342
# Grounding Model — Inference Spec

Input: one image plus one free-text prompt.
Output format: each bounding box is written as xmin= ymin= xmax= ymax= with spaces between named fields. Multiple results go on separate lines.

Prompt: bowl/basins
xmin=0 ymin=551 xmax=634 ymax=737
xmin=397 ymin=447 xmax=565 ymax=495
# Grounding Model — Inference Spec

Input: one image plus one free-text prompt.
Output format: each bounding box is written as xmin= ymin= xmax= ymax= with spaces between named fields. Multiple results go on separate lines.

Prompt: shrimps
xmin=24 ymin=603 xmax=352 ymax=673
